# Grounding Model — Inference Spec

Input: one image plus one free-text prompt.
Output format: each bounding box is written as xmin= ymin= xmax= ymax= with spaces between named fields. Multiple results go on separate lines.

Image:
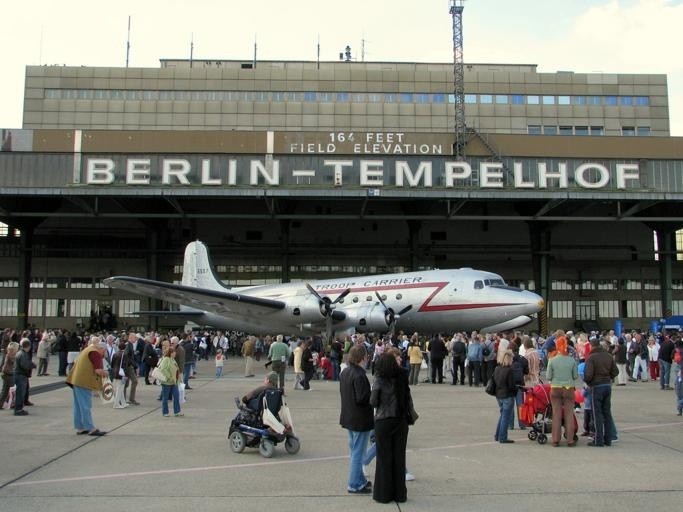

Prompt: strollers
xmin=513 ymin=383 xmax=580 ymax=446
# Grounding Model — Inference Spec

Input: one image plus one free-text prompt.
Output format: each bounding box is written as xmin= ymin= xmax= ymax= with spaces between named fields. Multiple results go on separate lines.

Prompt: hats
xmin=566 ymin=330 xmax=573 ymax=336
xmin=266 ymin=370 xmax=278 ymax=384
xmin=542 ymin=335 xmax=567 ymax=352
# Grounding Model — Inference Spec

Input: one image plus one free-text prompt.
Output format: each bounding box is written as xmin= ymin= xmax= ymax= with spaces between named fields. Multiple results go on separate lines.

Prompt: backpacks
xmin=481 ymin=342 xmax=492 ymax=356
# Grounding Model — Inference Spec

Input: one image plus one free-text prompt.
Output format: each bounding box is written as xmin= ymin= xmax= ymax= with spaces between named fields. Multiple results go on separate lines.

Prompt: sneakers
xmin=35 ymin=371 xmax=68 ymax=377
xmin=361 ymin=464 xmax=369 ymax=477
xmin=581 ymin=431 xmax=618 ymax=447
xmin=567 ymin=441 xmax=576 ymax=447
xmin=615 ymin=377 xmax=674 ymax=390
xmin=113 ymin=357 xmax=272 ymax=418
xmin=405 ymin=471 xmax=415 ymax=481
xmin=550 ymin=439 xmax=560 ymax=447
xmin=293 ymin=382 xmax=310 ymax=390
xmin=23 ymin=401 xmax=34 ymax=406
xmin=408 ymin=380 xmax=487 ymax=387
xmin=347 ymin=480 xmax=372 ymax=494
xmin=494 ymin=425 xmax=527 ymax=443
xmin=13 ymin=409 xmax=28 ymax=416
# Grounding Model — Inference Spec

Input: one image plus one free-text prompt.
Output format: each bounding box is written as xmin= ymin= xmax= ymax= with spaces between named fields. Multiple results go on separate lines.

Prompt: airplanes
xmin=103 ymin=238 xmax=545 ymax=344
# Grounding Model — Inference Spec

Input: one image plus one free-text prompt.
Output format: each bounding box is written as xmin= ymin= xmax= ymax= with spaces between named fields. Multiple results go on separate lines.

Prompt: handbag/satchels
xmin=261 ymin=396 xmax=285 ymax=434
xmin=98 ymin=375 xmax=115 ymax=405
xmin=118 ymin=366 xmax=127 ymax=376
xmin=288 ymin=352 xmax=294 ymax=366
xmin=277 ymin=395 xmax=294 ymax=434
xmin=151 ymin=367 xmax=167 ymax=382
xmin=2 ymin=386 xmax=16 ymax=409
xmin=517 ymin=392 xmax=536 ymax=427
xmin=485 ymin=377 xmax=496 ymax=396
xmin=172 ymin=383 xmax=186 ymax=406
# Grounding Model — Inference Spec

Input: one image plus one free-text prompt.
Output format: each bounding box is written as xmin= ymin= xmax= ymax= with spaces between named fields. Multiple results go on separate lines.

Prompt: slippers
xmin=76 ymin=428 xmax=105 ymax=436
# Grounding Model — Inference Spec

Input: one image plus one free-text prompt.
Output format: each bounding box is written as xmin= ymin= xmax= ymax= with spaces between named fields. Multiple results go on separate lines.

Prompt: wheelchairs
xmin=228 ymin=388 xmax=300 ymax=458
